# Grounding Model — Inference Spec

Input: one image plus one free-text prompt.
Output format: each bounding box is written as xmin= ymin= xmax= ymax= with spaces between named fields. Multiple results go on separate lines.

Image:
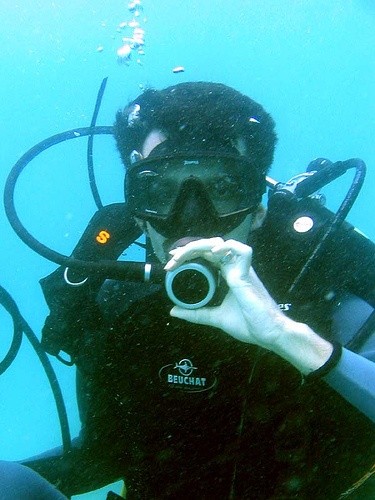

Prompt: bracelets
xmin=304 ymin=338 xmax=342 ymax=382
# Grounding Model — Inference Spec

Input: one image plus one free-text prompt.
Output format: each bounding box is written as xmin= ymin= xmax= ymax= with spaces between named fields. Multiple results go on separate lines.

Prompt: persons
xmin=2 ymin=77 xmax=374 ymax=500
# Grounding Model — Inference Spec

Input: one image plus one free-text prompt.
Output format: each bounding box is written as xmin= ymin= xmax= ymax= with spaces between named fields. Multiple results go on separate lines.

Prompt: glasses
xmin=123 ymin=150 xmax=267 ymax=221
